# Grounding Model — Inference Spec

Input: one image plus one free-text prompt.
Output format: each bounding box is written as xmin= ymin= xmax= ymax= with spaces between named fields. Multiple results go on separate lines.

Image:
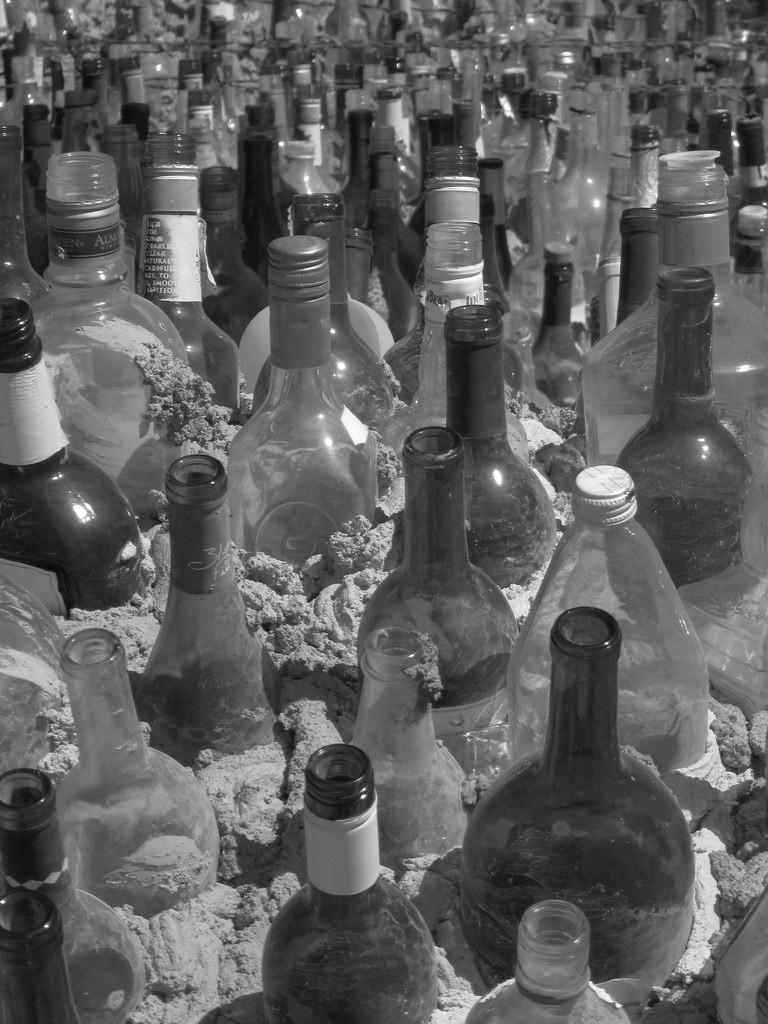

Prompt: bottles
xmin=0 ymin=0 xmax=768 ymax=1022
